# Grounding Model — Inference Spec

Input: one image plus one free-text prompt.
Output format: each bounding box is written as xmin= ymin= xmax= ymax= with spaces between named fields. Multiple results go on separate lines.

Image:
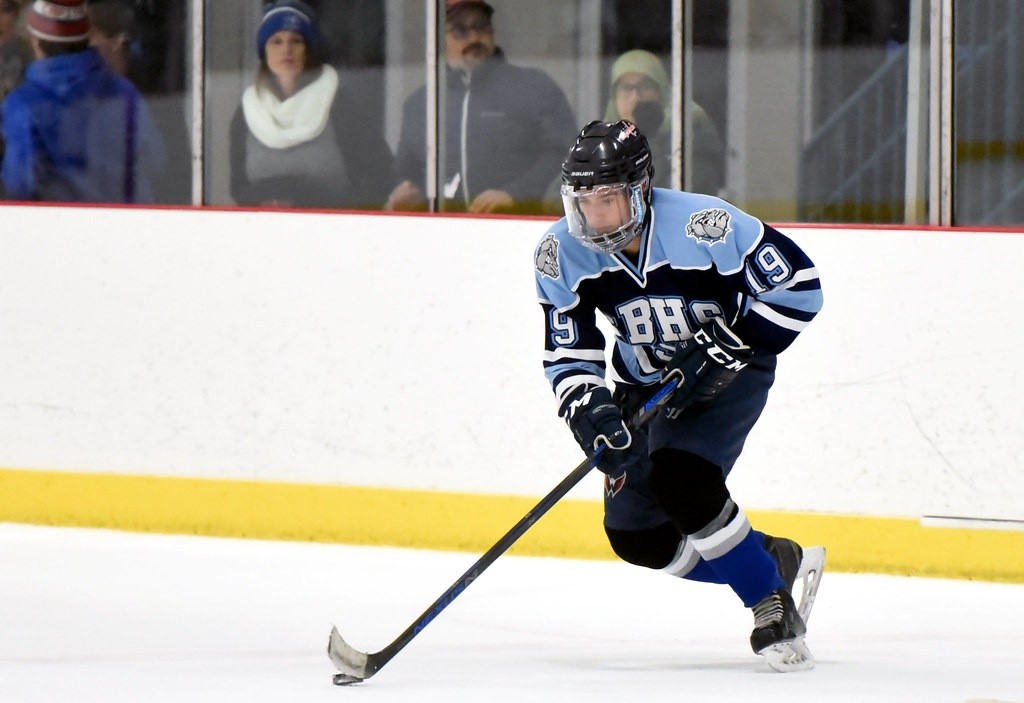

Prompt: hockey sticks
xmin=324 ymin=374 xmax=682 ymax=681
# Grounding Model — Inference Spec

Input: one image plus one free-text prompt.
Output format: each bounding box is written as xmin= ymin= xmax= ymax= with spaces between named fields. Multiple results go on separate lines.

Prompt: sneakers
xmin=749 ymin=588 xmax=815 ymax=673
xmin=755 ymin=531 xmax=825 ymax=626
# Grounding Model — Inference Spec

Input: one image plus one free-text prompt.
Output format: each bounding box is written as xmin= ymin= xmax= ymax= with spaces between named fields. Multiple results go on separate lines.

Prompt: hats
xmin=256 ymin=6 xmax=317 ymax=50
xmin=446 ymin=0 xmax=494 ymax=14
xmin=611 ymin=49 xmax=668 ymax=91
xmin=25 ymin=0 xmax=93 ymax=42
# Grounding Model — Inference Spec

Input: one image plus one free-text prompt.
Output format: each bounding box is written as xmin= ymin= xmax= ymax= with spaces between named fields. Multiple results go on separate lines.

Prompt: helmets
xmin=560 ymin=119 xmax=655 ymax=254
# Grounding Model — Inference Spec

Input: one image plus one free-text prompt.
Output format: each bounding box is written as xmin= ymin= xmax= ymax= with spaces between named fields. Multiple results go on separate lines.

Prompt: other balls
xmin=332 ymin=672 xmax=364 ymax=686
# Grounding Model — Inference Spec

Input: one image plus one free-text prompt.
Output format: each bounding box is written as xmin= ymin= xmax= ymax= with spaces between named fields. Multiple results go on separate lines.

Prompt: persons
xmin=532 ymin=119 xmax=827 ymax=672
xmin=543 ymin=48 xmax=718 ymax=218
xmin=230 ymin=0 xmax=393 ymax=209
xmin=383 ymin=1 xmax=579 ymax=216
xmin=0 ymin=0 xmax=168 ymax=206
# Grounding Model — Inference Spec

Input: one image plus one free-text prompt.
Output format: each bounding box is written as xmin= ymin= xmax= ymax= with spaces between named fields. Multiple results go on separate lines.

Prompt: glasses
xmin=446 ymin=19 xmax=492 ymax=37
xmin=617 ymin=80 xmax=658 ymax=97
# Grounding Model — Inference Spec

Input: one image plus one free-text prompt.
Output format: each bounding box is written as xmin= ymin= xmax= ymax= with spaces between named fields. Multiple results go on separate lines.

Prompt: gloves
xmin=557 ymin=383 xmax=638 ymax=475
xmin=657 ymin=315 xmax=755 ymax=425
